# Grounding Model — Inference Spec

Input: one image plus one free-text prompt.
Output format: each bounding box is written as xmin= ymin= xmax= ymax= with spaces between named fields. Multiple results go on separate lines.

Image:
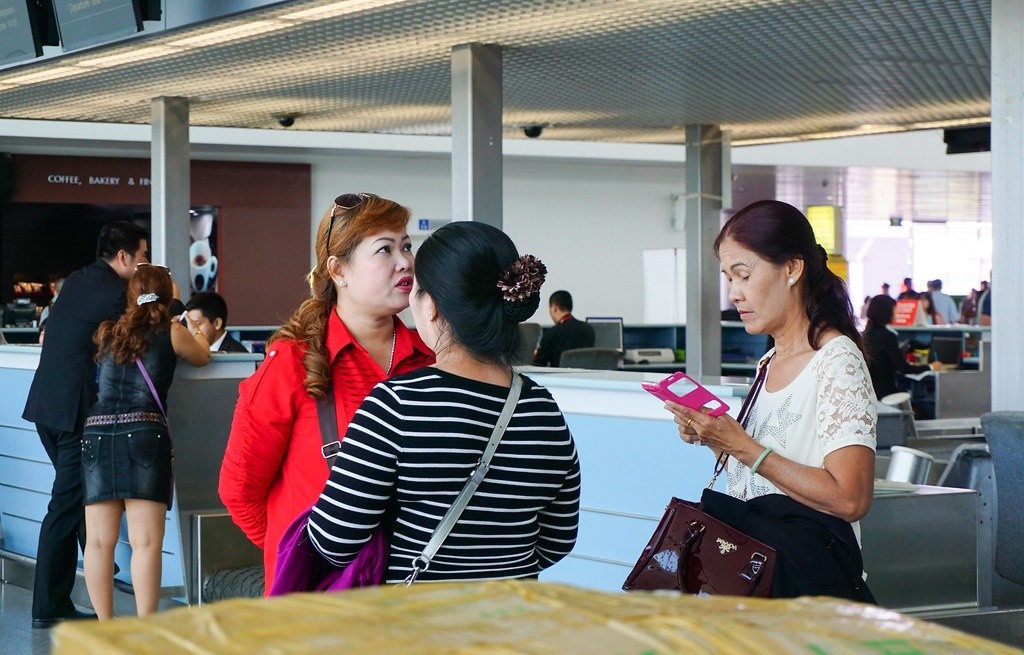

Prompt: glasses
xmin=327 ymin=193 xmax=379 ymax=256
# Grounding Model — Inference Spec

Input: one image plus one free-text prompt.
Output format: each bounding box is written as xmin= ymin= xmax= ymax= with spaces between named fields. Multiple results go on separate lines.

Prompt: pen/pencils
xmin=179 ymin=310 xmax=188 ymax=322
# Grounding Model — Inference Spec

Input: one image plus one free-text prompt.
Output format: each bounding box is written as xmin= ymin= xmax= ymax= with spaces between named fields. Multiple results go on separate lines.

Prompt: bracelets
xmin=751 ymin=447 xmax=773 ymax=474
xmin=193 ymin=330 xmax=203 ymax=337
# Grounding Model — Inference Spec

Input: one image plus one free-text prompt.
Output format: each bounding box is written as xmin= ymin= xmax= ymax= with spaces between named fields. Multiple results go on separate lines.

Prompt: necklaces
xmin=387 ymin=328 xmax=396 ymax=376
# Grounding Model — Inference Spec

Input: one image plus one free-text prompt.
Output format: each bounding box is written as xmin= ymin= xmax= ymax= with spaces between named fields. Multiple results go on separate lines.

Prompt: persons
xmin=217 ymin=192 xmax=436 ymax=597
xmin=859 ymin=271 xmax=991 ymax=420
xmin=664 ymin=200 xmax=878 ymax=596
xmin=530 ymin=290 xmax=596 ymax=370
xmin=310 ymin=221 xmax=581 ymax=587
xmin=20 ymin=220 xmax=258 ymax=629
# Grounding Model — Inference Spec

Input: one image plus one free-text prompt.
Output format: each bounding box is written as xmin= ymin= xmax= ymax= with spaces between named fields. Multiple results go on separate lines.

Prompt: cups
xmin=189 ymin=241 xmax=218 ymax=294
xmin=915 ymin=349 xmax=929 ymax=366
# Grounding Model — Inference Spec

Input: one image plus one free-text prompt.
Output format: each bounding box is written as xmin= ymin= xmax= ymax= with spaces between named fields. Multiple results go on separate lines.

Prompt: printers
xmin=624 ymin=348 xmax=675 ymax=364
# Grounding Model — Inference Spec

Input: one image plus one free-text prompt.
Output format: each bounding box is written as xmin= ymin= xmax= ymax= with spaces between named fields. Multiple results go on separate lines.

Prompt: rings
xmin=688 ymin=419 xmax=691 ymax=425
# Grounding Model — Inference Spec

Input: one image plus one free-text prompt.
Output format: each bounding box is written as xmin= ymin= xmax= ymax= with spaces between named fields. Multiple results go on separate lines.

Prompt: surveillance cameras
xmin=279 ymin=115 xmax=294 ymax=127
xmin=523 ymin=126 xmax=542 ymax=138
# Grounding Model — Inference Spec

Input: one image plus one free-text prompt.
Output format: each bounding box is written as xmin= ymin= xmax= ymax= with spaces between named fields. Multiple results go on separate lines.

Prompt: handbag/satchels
xmin=622 ymin=497 xmax=776 ymax=599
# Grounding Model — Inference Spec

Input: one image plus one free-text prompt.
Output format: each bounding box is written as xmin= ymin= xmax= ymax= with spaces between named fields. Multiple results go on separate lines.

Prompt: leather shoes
xmin=32 ymin=610 xmax=98 ymax=629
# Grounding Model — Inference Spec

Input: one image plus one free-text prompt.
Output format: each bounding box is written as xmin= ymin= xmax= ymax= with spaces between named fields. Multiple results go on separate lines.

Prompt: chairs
xmin=558 ymin=347 xmax=622 ymax=370
xmin=980 ymin=410 xmax=1024 ymax=587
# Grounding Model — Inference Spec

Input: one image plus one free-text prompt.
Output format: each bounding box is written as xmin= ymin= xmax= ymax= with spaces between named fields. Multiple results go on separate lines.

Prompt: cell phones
xmin=641 ymin=372 xmax=730 ymax=417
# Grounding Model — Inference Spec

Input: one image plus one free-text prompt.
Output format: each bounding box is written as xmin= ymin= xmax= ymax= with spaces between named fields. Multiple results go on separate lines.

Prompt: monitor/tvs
xmin=928 ymin=330 xmax=966 ymax=365
xmin=585 ymin=317 xmax=624 ymax=354
